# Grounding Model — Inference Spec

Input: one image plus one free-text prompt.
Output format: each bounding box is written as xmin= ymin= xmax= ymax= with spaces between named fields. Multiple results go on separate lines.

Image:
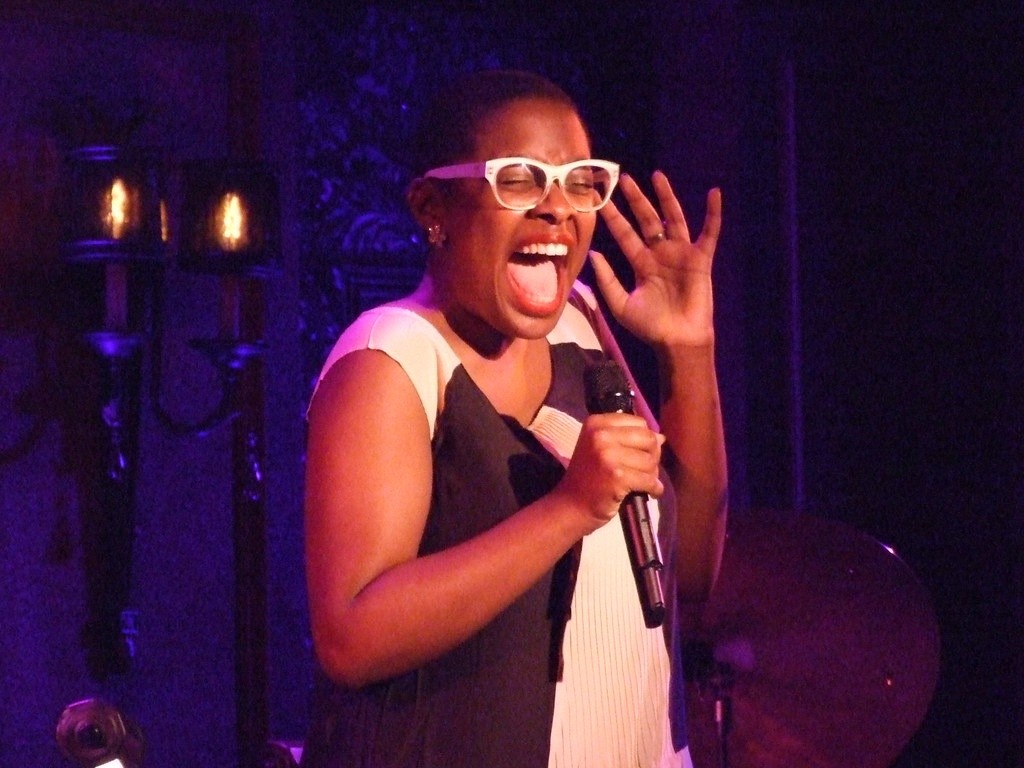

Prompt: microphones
xmin=582 ymin=361 xmax=668 ymax=631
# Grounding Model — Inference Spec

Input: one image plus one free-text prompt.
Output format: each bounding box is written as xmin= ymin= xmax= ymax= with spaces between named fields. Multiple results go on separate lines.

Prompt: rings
xmin=645 ymin=233 xmax=665 ymax=246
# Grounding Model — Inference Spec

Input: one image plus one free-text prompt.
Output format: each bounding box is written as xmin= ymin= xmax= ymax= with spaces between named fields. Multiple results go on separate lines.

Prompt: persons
xmin=303 ymin=71 xmax=728 ymax=768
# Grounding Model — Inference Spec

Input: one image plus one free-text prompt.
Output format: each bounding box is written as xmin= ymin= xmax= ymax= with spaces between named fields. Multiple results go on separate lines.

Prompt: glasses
xmin=422 ymin=156 xmax=621 ymax=212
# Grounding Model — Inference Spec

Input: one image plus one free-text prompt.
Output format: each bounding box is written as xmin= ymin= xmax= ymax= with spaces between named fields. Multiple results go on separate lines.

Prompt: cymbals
xmin=681 ymin=508 xmax=942 ymax=768
